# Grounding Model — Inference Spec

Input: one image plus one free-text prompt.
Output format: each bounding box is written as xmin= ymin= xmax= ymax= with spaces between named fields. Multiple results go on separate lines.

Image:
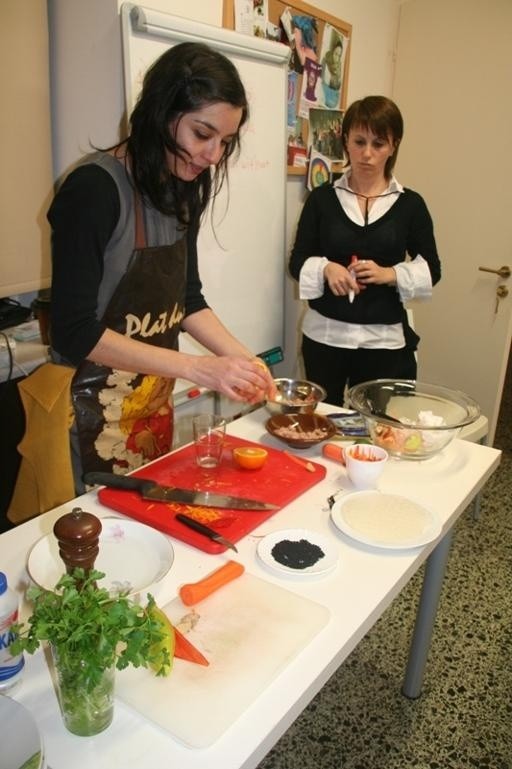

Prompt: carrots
xmin=322 ymin=443 xmax=344 ymax=464
xmin=179 ymin=559 xmax=245 ymax=607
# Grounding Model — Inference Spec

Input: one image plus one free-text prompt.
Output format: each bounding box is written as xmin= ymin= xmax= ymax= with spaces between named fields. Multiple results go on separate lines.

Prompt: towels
xmin=6 ymin=361 xmax=78 ymax=526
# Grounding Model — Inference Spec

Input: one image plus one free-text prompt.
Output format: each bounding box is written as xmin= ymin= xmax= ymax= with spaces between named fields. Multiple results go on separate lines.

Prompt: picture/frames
xmin=223 ymin=0 xmax=353 ymax=177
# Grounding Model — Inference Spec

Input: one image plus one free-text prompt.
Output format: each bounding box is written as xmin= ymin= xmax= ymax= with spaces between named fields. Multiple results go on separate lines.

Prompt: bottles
xmin=0 ymin=571 xmax=27 ymax=691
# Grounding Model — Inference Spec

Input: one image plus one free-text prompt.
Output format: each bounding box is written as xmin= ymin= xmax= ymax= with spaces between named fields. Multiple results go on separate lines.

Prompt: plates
xmin=330 ymin=489 xmax=443 ymax=550
xmin=256 ymin=527 xmax=340 ymax=574
xmin=324 ymin=410 xmax=371 ymax=437
xmin=27 ymin=518 xmax=175 ymax=603
xmin=0 ymin=692 xmax=45 ymax=769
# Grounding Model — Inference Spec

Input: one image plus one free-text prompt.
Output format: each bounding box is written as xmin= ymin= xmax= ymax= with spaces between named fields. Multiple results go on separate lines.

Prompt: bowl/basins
xmin=261 ymin=377 xmax=328 ymax=417
xmin=264 ymin=413 xmax=337 ymax=449
xmin=346 ymin=378 xmax=482 ymax=461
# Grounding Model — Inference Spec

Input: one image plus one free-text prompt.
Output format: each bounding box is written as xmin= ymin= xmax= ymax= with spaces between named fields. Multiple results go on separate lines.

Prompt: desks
xmin=0 ymin=398 xmax=502 ymax=768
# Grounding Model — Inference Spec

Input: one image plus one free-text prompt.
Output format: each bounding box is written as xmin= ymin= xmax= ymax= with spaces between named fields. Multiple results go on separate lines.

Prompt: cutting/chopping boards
xmin=97 ymin=430 xmax=327 ymax=555
xmin=113 ymin=566 xmax=334 ymax=753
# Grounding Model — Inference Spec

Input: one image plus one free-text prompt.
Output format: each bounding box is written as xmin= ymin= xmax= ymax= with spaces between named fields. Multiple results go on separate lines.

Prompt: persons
xmin=47 ymin=42 xmax=277 ymax=527
xmin=323 ymin=30 xmax=342 ymax=91
xmin=288 ymin=95 xmax=441 ymax=411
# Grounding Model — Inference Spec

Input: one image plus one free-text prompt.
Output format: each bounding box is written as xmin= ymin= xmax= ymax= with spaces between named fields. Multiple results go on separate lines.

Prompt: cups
xmin=44 ymin=640 xmax=117 ymax=738
xmin=192 ymin=413 xmax=226 ymax=469
xmin=342 ymin=443 xmax=390 ymax=488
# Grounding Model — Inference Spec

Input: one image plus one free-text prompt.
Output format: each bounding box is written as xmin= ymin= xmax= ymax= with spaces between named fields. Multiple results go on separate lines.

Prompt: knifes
xmin=82 ymin=471 xmax=281 ymax=511
xmin=175 ymin=513 xmax=239 ymax=554
